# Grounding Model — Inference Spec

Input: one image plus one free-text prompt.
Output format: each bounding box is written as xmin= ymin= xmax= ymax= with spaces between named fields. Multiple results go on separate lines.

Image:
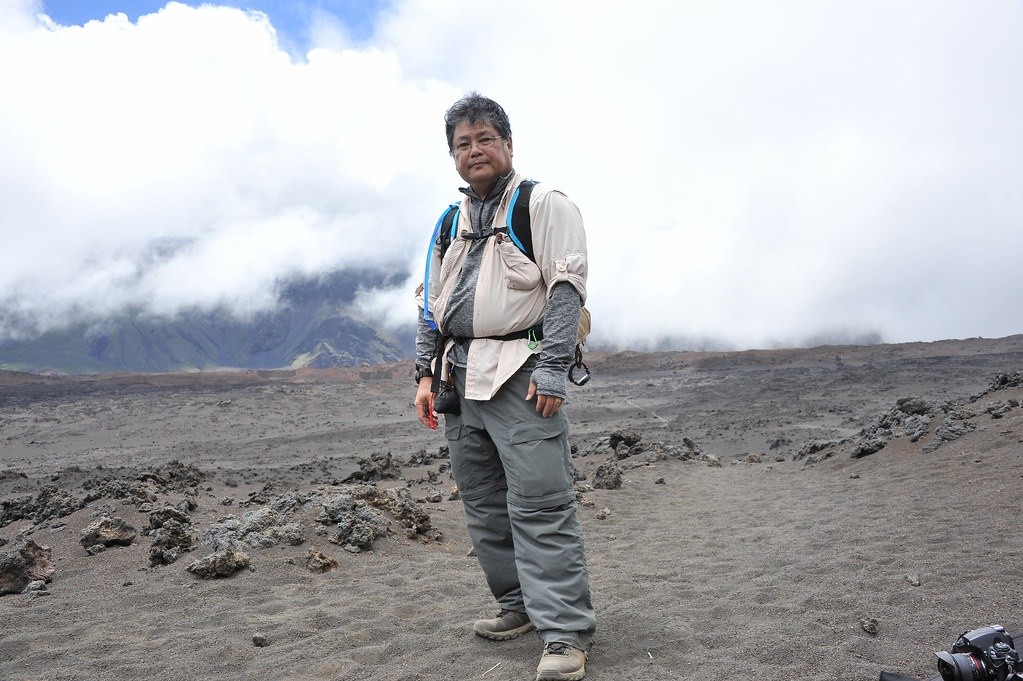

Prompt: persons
xmin=413 ymin=91 xmax=597 ymax=681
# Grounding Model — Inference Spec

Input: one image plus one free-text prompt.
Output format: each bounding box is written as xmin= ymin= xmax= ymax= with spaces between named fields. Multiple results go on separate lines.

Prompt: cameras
xmin=934 ymin=625 xmax=1023 ymax=681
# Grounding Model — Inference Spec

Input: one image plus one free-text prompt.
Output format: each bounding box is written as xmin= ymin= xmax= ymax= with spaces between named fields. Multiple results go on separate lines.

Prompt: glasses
xmin=451 ymin=135 xmax=502 ymax=155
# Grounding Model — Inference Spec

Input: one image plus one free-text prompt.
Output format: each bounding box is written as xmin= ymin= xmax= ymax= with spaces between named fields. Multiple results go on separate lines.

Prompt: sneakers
xmin=537 ymin=642 xmax=589 ymax=681
xmin=473 ymin=608 xmax=534 ymax=640
